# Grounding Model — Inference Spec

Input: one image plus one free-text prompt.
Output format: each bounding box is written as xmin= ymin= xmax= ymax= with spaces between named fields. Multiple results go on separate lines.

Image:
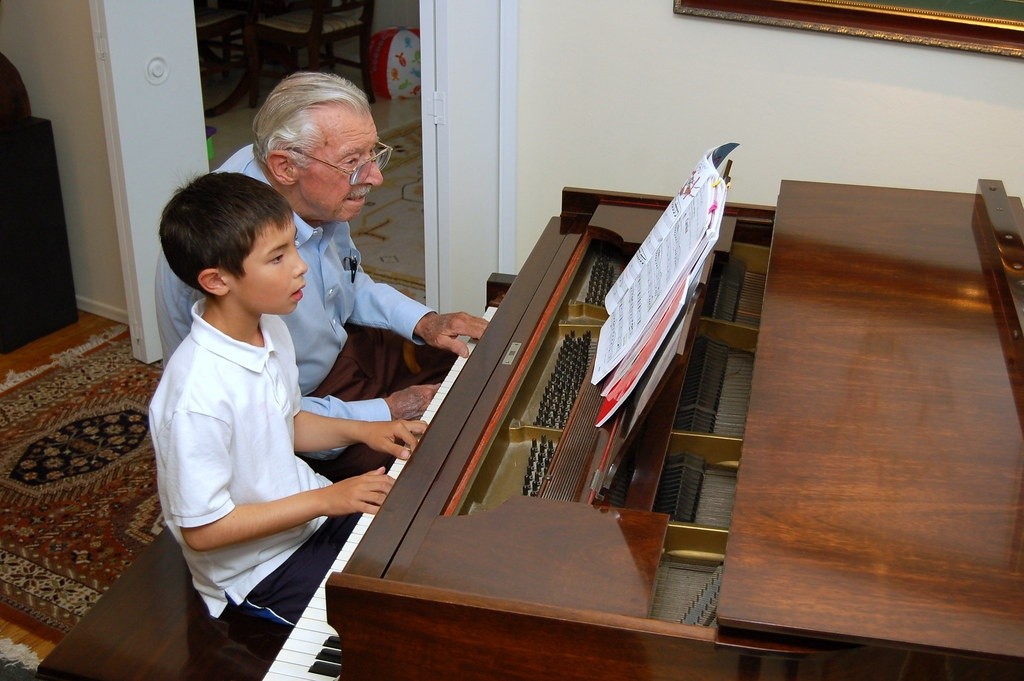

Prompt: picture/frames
xmin=670 ymin=0 xmax=1024 ymax=60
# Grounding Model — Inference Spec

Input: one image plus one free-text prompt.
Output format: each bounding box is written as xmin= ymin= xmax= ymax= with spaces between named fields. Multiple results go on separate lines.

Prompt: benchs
xmin=35 ymin=529 xmax=290 ymax=681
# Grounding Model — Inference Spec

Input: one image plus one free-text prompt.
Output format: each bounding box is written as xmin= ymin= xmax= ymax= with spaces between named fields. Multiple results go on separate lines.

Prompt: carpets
xmin=344 ymin=117 xmax=427 ymax=308
xmin=0 ymin=324 xmax=163 ymax=643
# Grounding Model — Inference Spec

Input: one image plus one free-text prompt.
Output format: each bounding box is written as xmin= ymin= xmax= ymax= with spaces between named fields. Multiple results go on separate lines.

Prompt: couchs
xmin=0 ymin=53 xmax=81 ymax=353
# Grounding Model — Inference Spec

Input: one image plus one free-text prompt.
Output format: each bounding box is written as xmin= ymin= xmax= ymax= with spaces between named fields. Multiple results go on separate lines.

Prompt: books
xmin=592 ymin=139 xmax=744 ymax=428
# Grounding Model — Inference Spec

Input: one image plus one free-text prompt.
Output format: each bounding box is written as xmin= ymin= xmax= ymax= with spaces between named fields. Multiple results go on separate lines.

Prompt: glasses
xmin=285 ymin=142 xmax=393 ymax=185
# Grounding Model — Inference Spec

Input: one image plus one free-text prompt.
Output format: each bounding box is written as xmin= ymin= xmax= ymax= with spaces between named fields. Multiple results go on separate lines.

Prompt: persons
xmin=149 ymin=171 xmax=428 ymax=661
xmin=156 ymin=72 xmax=498 ymax=482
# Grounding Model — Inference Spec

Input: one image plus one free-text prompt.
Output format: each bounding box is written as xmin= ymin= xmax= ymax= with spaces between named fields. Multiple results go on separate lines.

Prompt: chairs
xmin=193 ymin=0 xmax=376 ymax=103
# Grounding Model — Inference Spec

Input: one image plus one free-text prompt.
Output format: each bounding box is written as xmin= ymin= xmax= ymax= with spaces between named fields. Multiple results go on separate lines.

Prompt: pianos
xmin=259 ymin=177 xmax=1024 ymax=681
